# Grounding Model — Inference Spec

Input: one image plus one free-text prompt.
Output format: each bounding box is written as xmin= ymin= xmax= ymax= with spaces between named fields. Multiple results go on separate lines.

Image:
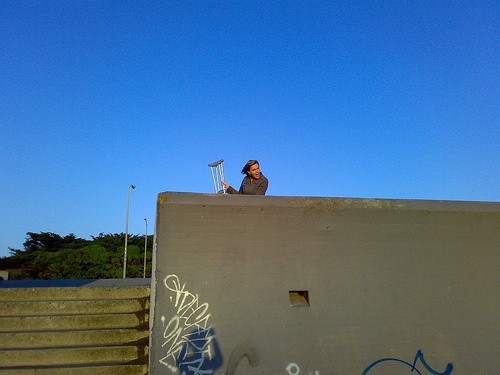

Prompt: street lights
xmin=123 ymin=184 xmax=137 ymax=279
xmin=143 ymin=218 xmax=147 ymax=279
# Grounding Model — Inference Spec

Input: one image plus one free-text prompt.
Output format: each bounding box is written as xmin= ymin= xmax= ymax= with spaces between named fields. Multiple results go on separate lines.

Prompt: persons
xmin=220 ymin=159 xmax=269 ymax=196
